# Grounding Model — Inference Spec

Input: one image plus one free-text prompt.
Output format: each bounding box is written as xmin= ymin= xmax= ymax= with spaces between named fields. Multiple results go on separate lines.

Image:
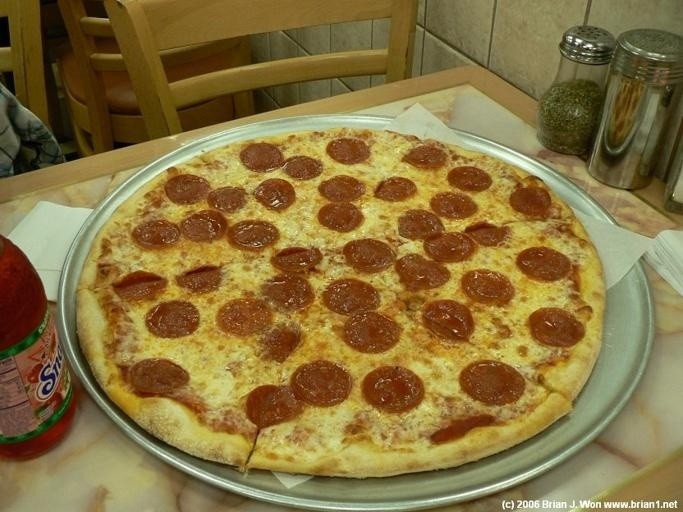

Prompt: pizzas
xmin=74 ymin=125 xmax=607 ymax=477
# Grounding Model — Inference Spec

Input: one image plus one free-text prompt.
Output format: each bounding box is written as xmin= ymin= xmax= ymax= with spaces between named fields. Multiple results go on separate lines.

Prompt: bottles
xmin=585 ymin=27 xmax=682 ymax=194
xmin=533 ymin=23 xmax=620 ymax=159
xmin=0 ymin=233 xmax=81 ymax=464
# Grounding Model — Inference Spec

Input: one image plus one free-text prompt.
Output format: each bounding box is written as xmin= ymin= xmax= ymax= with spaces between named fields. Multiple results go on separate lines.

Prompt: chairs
xmin=104 ymin=0 xmax=418 ymax=139
xmin=58 ymin=0 xmax=254 ymax=157
xmin=0 ymin=1 xmax=52 ymax=134
